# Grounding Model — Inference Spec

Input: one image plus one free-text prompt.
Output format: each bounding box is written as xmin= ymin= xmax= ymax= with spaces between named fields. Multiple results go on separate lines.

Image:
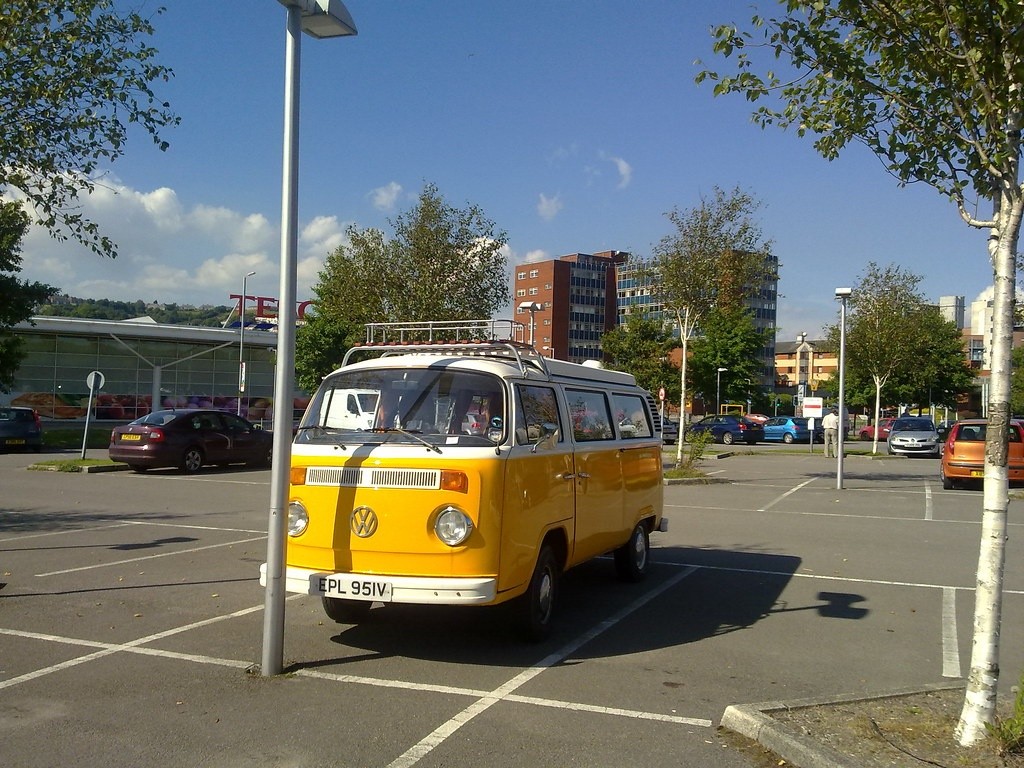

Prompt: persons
xmin=901 ymin=408 xmax=911 ymax=418
xmin=822 ymin=409 xmax=840 ymax=458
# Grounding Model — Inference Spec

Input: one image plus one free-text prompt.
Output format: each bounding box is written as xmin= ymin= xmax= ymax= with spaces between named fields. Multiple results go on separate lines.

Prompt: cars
xmin=0 ymin=406 xmax=43 ymax=454
xmin=110 ymin=410 xmax=272 ymax=473
xmin=860 ymin=417 xmax=1024 ymax=490
xmin=660 ymin=414 xmax=817 ymax=445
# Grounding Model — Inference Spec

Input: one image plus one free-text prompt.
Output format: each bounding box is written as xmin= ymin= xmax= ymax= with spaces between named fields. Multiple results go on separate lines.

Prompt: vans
xmin=260 ymin=320 xmax=667 ymax=642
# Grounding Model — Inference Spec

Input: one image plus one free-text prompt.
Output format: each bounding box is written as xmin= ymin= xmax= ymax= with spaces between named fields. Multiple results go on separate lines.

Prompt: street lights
xmin=518 ymin=301 xmax=545 ymax=346
xmin=258 ymin=1 xmax=358 ymax=676
xmin=716 ymin=368 xmax=728 ymax=415
xmin=744 ymin=379 xmax=751 ymax=414
xmin=238 ymin=272 xmax=256 ymax=416
xmin=833 ymin=287 xmax=853 ymax=491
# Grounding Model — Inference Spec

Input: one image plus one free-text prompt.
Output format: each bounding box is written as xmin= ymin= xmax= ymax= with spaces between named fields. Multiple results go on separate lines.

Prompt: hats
xmin=831 ymin=408 xmax=838 ymax=414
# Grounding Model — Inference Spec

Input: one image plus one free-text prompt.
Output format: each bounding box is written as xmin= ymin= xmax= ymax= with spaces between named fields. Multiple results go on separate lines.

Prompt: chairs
xmin=964 ymin=428 xmax=976 ymax=439
xmin=483 ymin=392 xmax=503 ymax=439
xmin=399 ymin=390 xmax=436 ymax=433
